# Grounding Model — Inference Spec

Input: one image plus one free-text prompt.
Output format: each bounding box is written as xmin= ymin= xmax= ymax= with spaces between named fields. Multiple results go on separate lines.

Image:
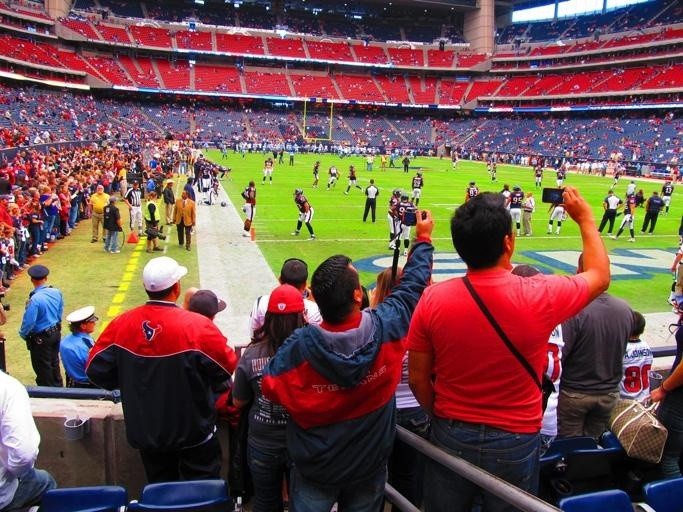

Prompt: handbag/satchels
xmin=608 ymin=395 xmax=668 ymax=463
xmin=228 ymin=410 xmax=254 ymax=504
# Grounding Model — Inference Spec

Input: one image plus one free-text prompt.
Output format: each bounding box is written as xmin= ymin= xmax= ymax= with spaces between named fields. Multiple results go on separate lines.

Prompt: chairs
xmin=126 ymin=478 xmax=244 ymax=512
xmin=27 ymin=484 xmax=129 ymax=512
xmin=565 ymin=446 xmax=620 ymax=494
xmin=643 ymin=477 xmax=683 ymax=512
xmin=547 ymin=436 xmax=597 ymax=462
xmin=601 ymin=431 xmax=619 ymax=448
xmin=537 ymin=453 xmax=560 ymax=473
xmin=557 ymin=489 xmax=636 ymax=512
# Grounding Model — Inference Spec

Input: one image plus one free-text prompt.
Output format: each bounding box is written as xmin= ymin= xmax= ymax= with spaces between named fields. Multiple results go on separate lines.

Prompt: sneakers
xmin=388 ymin=245 xmax=408 ymax=256
xmin=138 ymin=231 xmax=148 ymax=236
xmin=0 ymin=274 xmax=18 ymax=292
xmin=242 ymin=229 xmax=252 ymax=236
xmin=90 ymin=238 xmax=120 ymax=254
xmin=146 ymin=247 xmax=164 ymax=253
xmin=291 ymin=230 xmax=315 ymax=240
xmin=179 ymin=244 xmax=191 ymax=251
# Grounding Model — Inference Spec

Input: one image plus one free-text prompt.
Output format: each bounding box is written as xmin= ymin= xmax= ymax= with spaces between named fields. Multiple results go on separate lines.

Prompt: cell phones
xmin=542 ymin=188 xmax=564 ymax=204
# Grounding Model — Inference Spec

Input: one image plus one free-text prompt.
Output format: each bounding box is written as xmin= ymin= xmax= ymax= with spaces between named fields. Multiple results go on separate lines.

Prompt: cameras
xmin=402 ymin=207 xmax=427 ymax=226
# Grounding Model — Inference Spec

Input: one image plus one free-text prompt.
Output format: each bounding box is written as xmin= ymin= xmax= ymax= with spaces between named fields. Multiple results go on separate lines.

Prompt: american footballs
xmin=221 ymin=202 xmax=226 ymax=206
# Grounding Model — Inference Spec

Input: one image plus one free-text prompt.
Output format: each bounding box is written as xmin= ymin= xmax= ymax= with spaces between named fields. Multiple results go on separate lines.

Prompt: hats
xmin=109 ymin=197 xmax=116 ymax=202
xmin=165 ymin=179 xmax=174 ymax=185
xmin=134 ymin=180 xmax=139 ymax=184
xmin=143 ymin=257 xmax=226 ymax=316
xmin=267 ymin=282 xmax=304 ymax=315
xmin=66 ymin=306 xmax=98 ymax=322
xmin=28 ymin=265 xmax=49 ymax=280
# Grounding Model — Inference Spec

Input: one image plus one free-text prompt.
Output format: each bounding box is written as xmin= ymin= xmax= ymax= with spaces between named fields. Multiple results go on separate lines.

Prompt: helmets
xmin=393 ymin=188 xmax=408 ymax=201
xmin=295 ymin=189 xmax=303 ymax=195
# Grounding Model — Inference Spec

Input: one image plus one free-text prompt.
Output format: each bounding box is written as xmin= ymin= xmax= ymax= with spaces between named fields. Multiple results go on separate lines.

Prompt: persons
xmin=233 ymin=283 xmax=310 ymax=512
xmin=85 ymin=257 xmax=239 ymax=484
xmin=1 ymin=0 xmax=682 ymax=478
xmin=261 ymin=210 xmax=434 ymax=512
xmin=408 ymin=185 xmax=610 ymax=512
xmin=0 ymin=369 xmax=58 ymax=512
